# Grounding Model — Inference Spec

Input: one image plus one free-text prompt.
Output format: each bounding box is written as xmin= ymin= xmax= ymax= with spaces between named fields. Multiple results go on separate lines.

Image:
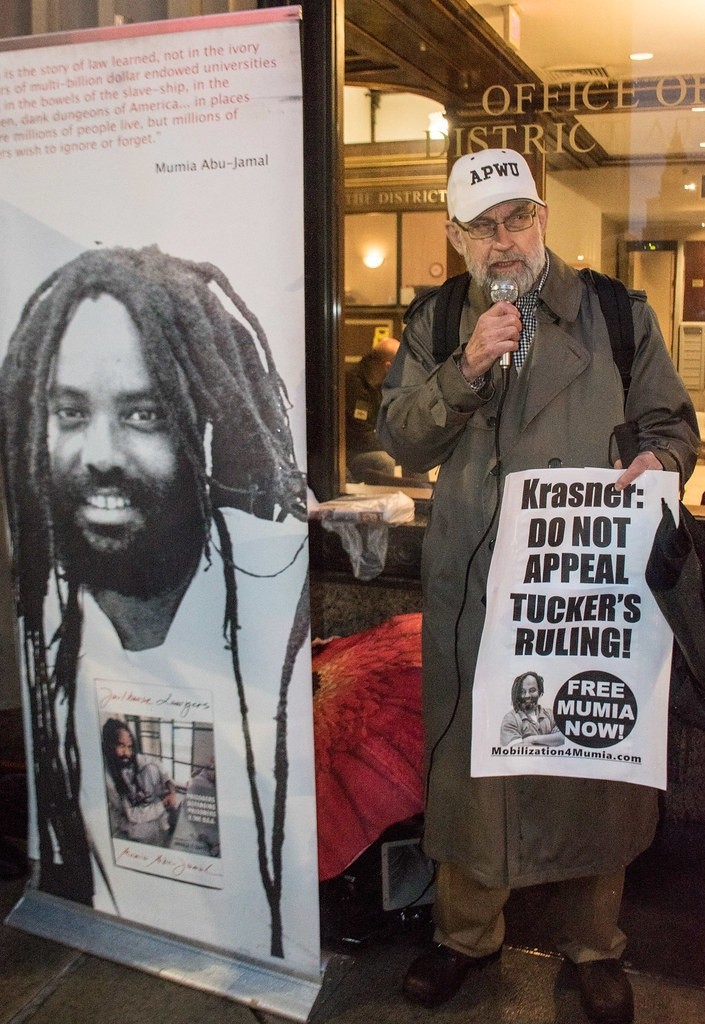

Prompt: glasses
xmin=451 ymin=204 xmax=539 ymax=240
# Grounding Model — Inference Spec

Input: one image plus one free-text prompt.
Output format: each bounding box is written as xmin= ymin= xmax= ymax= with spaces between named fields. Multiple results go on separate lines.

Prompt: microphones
xmin=490 ymin=277 xmax=518 ymax=369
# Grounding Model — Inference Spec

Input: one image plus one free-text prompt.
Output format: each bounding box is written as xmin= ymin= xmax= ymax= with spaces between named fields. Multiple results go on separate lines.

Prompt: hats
xmin=446 ymin=148 xmax=548 ymax=224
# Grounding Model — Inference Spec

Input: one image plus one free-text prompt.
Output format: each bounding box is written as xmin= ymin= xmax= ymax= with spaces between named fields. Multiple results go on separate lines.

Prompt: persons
xmin=375 ymin=148 xmax=702 ymax=1024
xmin=347 ymin=337 xmax=430 ymax=485
xmin=0 ymin=245 xmax=319 ymax=977
xmin=101 ymin=719 xmax=176 ymax=840
xmin=500 ymin=671 xmax=565 ymax=746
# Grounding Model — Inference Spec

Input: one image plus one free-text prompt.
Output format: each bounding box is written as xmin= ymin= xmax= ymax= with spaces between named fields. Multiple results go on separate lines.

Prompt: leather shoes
xmin=403 ymin=933 xmax=502 ymax=1005
xmin=574 ymin=951 xmax=634 ymax=1024
